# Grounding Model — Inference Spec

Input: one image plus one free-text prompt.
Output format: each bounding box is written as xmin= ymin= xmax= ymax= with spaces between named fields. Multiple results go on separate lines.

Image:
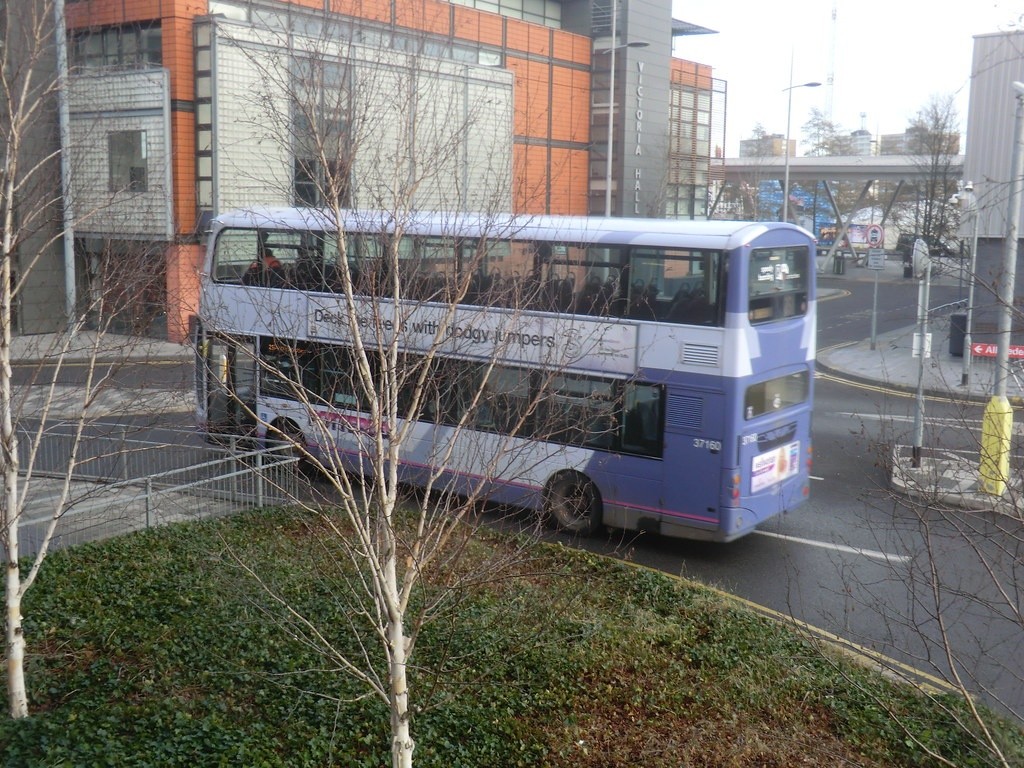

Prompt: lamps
xmin=964 ymin=180 xmax=975 ymax=192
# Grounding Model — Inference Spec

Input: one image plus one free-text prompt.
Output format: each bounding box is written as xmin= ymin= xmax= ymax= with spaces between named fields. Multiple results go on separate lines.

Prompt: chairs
xmin=638 ymin=277 xmax=659 ymax=320
xmin=664 ymin=282 xmax=692 ymax=323
xmin=626 ymin=279 xmax=646 ymax=320
xmin=686 ymin=280 xmax=707 ymax=322
xmin=370 ymin=266 xmax=617 ymax=316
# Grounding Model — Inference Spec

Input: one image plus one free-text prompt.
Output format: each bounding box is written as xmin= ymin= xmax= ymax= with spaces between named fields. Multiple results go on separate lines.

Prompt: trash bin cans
xmin=188 ymin=315 xmax=202 ymax=344
xmin=834 ymin=249 xmax=845 ymax=274
xmin=949 ymin=312 xmax=967 ymax=356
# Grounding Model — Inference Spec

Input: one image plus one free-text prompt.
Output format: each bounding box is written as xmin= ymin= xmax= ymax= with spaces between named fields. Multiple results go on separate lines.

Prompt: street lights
xmin=601 ymin=38 xmax=650 ymax=219
xmin=948 ymin=181 xmax=980 ymax=385
xmin=780 ymin=80 xmax=822 ymax=222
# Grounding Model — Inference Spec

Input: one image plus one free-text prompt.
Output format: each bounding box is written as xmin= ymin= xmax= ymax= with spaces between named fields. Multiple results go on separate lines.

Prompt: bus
xmin=188 ymin=207 xmax=819 ymax=543
